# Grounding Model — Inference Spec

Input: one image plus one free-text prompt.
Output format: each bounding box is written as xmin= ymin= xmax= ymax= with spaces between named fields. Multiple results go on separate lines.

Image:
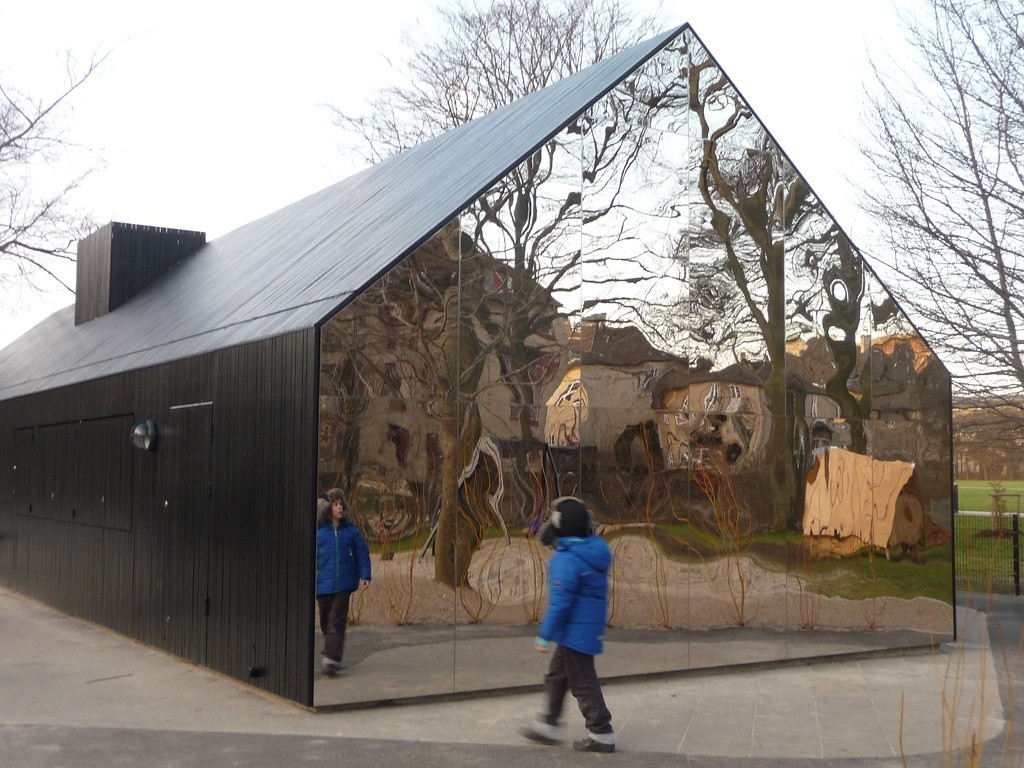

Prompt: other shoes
xmin=574 ymin=737 xmax=615 ymax=753
xmin=524 ymin=729 xmax=556 ymax=744
xmin=321 ymin=658 xmax=341 ymax=676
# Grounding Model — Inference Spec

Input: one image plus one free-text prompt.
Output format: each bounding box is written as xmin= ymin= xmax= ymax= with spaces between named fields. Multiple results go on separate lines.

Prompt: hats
xmin=317 ymin=488 xmax=350 ymax=522
xmin=540 ymin=496 xmax=596 ymax=547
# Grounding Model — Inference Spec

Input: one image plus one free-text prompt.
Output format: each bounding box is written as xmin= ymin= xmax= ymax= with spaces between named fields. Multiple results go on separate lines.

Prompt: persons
xmin=317 ymin=487 xmax=372 ymax=674
xmin=517 ymin=497 xmax=615 ymax=752
